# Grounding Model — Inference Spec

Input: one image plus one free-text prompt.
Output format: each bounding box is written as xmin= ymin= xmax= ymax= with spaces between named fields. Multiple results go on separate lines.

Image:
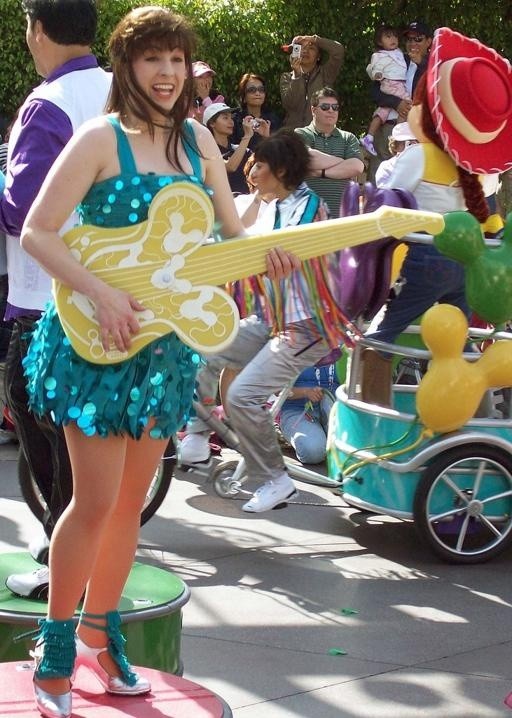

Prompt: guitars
xmin=53 ymin=182 xmax=445 ymax=365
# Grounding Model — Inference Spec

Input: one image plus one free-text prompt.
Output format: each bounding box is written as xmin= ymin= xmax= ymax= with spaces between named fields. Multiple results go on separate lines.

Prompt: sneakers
xmin=177 ymin=434 xmax=213 ymax=470
xmin=29 ymin=535 xmax=50 ymax=569
xmin=242 ymin=479 xmax=299 ymax=514
xmin=5 ymin=569 xmax=86 ymax=604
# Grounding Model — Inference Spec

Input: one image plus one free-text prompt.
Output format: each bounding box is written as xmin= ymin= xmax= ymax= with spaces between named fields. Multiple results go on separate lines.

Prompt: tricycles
xmin=16 ymin=322 xmax=512 ymax=567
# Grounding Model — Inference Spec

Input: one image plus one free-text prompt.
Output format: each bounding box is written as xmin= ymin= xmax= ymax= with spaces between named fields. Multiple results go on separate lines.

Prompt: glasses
xmin=404 ymin=35 xmax=423 ymax=43
xmin=317 ymin=103 xmax=339 ymax=111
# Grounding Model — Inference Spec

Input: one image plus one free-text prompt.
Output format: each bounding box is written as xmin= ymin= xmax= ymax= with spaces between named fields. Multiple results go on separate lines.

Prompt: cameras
xmin=249 ymin=118 xmax=261 ymax=130
xmin=291 ymin=44 xmax=301 ymax=59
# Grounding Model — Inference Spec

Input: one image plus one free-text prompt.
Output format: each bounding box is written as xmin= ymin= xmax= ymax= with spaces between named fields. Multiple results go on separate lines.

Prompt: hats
xmin=392 ymin=121 xmax=416 ymax=142
xmin=192 ymin=61 xmax=267 ymax=129
xmin=402 ymin=22 xmax=430 ymax=36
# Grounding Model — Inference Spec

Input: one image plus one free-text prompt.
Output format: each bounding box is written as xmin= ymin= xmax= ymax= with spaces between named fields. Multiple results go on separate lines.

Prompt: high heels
xmin=72 ymin=615 xmax=151 ymax=695
xmin=33 ymin=617 xmax=77 ymax=718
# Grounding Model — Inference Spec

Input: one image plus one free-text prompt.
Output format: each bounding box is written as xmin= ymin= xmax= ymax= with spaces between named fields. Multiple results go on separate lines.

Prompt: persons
xmin=360 ymin=27 xmax=512 ymax=353
xmin=175 ymin=131 xmax=350 ymax=515
xmin=0 ymin=0 xmax=114 ymax=605
xmin=359 ymin=22 xmax=433 ymax=188
xmin=182 ymin=33 xmax=367 ymax=465
xmin=18 ymin=4 xmax=303 ymax=718
